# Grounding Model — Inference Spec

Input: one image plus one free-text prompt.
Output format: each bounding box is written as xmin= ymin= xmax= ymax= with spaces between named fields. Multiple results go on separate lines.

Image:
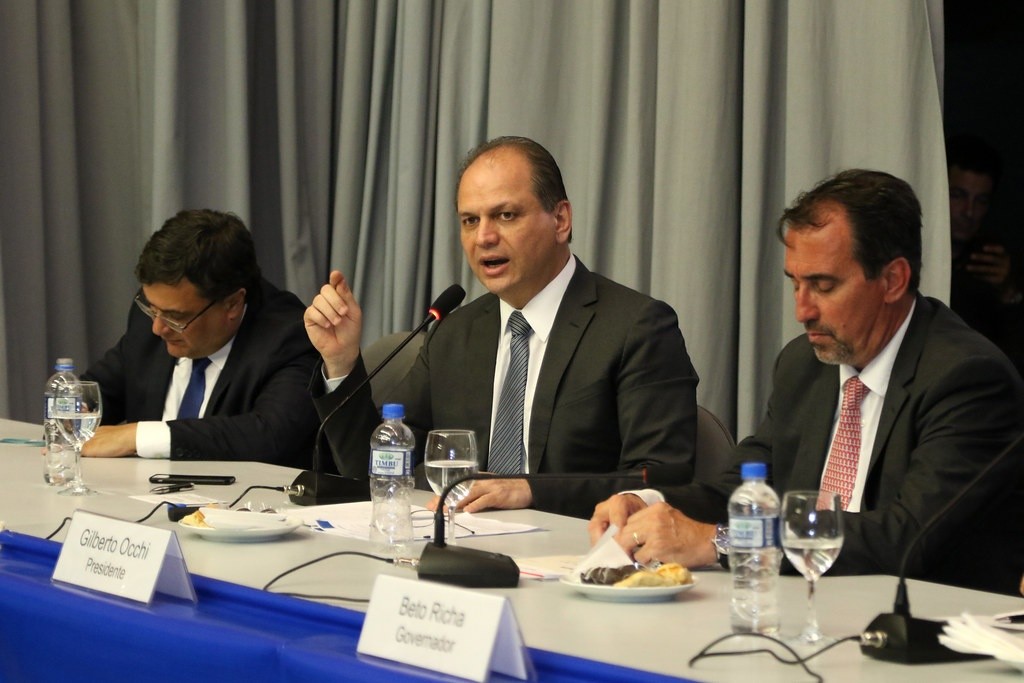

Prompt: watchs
xmin=711 ymin=522 xmax=729 ymax=570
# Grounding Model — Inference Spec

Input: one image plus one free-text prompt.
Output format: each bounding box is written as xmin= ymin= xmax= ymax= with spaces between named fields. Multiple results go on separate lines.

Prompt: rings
xmin=632 ymin=530 xmax=641 ymax=546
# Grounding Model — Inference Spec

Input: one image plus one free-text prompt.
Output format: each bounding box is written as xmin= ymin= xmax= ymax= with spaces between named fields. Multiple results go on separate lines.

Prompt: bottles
xmin=43 ymin=358 xmax=78 ymax=484
xmin=729 ymin=463 xmax=781 ymax=637
xmin=368 ymin=404 xmax=413 ymax=551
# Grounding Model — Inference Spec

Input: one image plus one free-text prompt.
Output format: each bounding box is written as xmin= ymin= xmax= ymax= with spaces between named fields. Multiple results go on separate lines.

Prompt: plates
xmin=561 ymin=573 xmax=696 ymax=601
xmin=179 ymin=510 xmax=302 ymax=542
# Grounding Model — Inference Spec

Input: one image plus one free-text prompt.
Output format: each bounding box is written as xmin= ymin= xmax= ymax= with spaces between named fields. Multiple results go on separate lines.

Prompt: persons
xmin=304 ymin=136 xmax=700 ymax=513
xmin=588 ymin=168 xmax=1024 ymax=577
xmin=40 ymin=208 xmax=318 ymax=477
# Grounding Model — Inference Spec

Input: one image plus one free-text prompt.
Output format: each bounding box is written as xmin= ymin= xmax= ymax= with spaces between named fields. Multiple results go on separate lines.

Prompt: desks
xmin=0 ymin=416 xmax=1024 ymax=683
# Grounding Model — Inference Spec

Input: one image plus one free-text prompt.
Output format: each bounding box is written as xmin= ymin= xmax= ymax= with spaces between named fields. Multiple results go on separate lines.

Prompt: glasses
xmin=134 ymin=292 xmax=216 ymax=334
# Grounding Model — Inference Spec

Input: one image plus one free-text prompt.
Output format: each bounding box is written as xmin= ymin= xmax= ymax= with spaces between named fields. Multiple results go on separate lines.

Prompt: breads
xmin=182 ymin=503 xmax=217 ymax=528
xmin=579 ymin=563 xmax=692 ymax=587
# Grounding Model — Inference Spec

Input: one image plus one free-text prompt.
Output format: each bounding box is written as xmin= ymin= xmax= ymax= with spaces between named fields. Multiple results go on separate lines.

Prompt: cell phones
xmin=150 ymin=474 xmax=236 ymax=485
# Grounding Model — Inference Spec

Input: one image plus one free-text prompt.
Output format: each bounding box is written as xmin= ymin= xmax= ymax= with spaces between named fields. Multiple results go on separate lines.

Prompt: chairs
xmin=362 ymin=329 xmax=425 ymax=407
xmin=690 ymin=406 xmax=740 ymax=488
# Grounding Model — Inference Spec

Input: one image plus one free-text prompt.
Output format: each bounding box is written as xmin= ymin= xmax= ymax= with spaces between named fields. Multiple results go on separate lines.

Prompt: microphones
xmin=288 ymin=284 xmax=466 ymax=506
xmin=416 ymin=463 xmax=693 ymax=588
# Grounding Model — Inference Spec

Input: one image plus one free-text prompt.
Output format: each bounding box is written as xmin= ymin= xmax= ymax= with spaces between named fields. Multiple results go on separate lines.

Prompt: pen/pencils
xmin=149 ymin=482 xmax=194 ymax=494
xmin=387 ymin=556 xmax=417 ymax=566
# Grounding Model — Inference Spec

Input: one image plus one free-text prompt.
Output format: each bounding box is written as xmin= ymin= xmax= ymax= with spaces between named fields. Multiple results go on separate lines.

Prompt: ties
xmin=176 ymin=358 xmax=212 ymax=420
xmin=815 ymin=376 xmax=870 ymax=511
xmin=488 ymin=311 xmax=532 ymax=474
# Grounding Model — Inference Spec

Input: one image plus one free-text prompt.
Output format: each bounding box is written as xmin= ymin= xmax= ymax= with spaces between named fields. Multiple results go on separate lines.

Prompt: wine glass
xmin=53 ymin=381 xmax=103 ymax=497
xmin=425 ymin=430 xmax=478 ymax=545
xmin=779 ymin=489 xmax=839 ymax=647
